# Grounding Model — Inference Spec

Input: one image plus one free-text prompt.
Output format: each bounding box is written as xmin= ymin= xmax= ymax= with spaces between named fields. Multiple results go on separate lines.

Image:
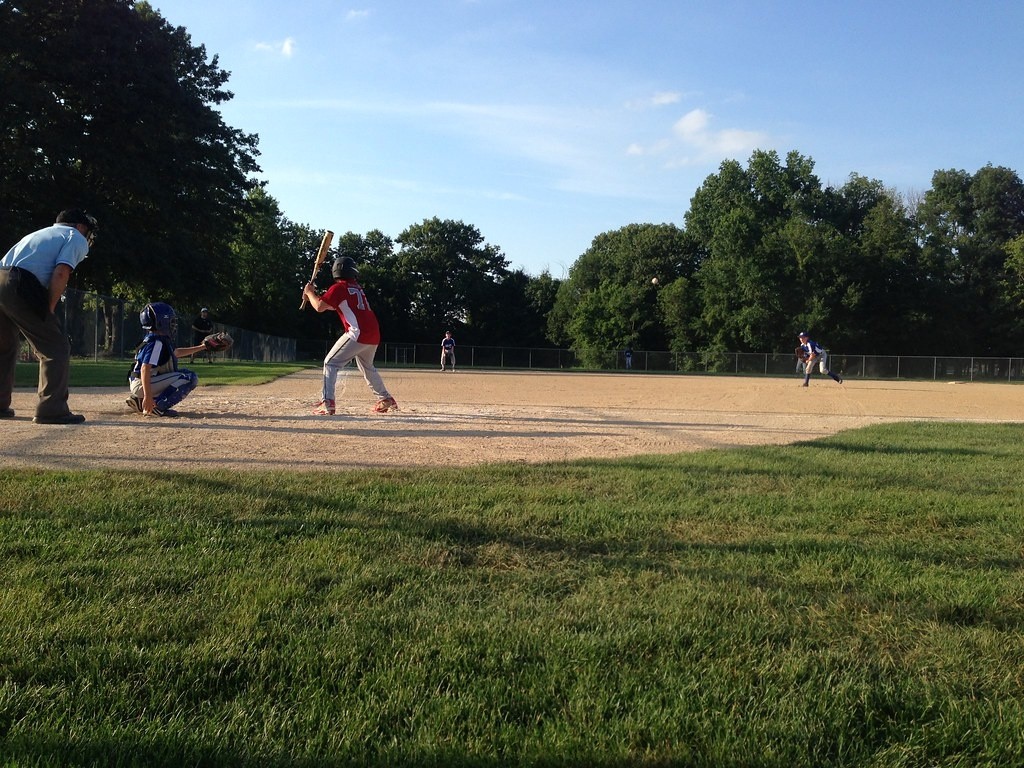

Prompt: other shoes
xmin=440 ymin=369 xmax=445 ymax=371
xmin=190 ymin=361 xmax=194 ymax=364
xmin=803 ymin=383 xmax=808 ymax=387
xmin=834 ymin=376 xmax=842 ymax=384
xmin=451 ymin=368 xmax=456 ymax=371
xmin=209 ymin=361 xmax=213 ymax=363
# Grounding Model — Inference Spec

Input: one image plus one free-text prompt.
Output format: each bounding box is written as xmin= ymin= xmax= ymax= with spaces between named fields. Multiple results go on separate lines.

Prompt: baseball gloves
xmin=201 ymin=331 xmax=235 ymax=353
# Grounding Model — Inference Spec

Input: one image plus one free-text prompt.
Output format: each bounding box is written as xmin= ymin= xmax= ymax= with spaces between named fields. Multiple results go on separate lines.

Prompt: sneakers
xmin=373 ymin=397 xmax=398 ymax=413
xmin=125 ymin=396 xmax=178 ymax=418
xmin=0 ymin=408 xmax=15 ymax=418
xmin=32 ymin=412 xmax=86 ymax=424
xmin=312 ymin=400 xmax=336 ymax=415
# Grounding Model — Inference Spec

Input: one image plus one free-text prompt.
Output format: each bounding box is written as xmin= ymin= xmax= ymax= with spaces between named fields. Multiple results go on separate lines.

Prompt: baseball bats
xmin=299 ymin=229 xmax=334 ymax=310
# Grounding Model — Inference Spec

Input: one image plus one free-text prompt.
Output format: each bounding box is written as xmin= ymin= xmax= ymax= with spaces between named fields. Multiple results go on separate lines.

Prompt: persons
xmin=127 ymin=301 xmax=230 ymax=417
xmin=624 ymin=346 xmax=632 ymax=369
xmin=302 ymin=257 xmax=398 ymax=415
xmin=190 ymin=308 xmax=213 ymax=364
xmin=439 ymin=331 xmax=455 ymax=372
xmin=795 ymin=332 xmax=843 ymax=386
xmin=0 ymin=208 xmax=99 ymax=424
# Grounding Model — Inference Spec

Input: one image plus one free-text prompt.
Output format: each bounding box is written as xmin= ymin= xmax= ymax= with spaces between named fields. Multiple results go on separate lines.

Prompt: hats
xmin=446 ymin=331 xmax=451 ymax=334
xmin=797 ymin=332 xmax=809 ymax=338
xmin=201 ymin=308 xmax=208 ymax=312
xmin=56 ymin=209 xmax=93 ymax=224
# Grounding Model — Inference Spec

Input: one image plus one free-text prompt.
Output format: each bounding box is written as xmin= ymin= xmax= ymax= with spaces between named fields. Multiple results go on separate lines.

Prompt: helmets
xmin=332 ymin=257 xmax=357 ymax=278
xmin=141 ymin=302 xmax=178 ymax=339
